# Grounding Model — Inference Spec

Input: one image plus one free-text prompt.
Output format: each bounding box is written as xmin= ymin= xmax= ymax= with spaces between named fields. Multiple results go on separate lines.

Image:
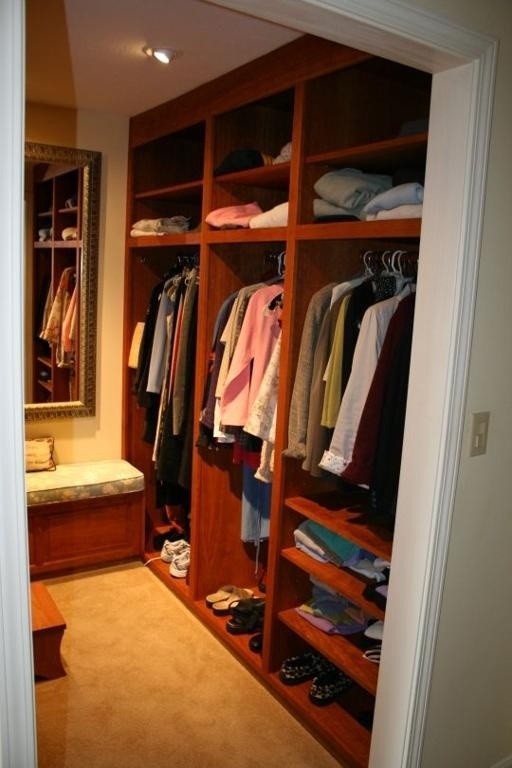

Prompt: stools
xmin=29 ymin=579 xmax=68 ymax=685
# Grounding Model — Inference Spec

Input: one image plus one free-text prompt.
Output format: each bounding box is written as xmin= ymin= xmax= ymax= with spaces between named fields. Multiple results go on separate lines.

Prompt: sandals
xmin=226 ymin=597 xmax=266 ymax=633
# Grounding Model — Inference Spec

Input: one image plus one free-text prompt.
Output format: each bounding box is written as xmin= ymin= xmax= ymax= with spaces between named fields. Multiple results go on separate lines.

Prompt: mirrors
xmin=24 ymin=141 xmax=104 ymax=423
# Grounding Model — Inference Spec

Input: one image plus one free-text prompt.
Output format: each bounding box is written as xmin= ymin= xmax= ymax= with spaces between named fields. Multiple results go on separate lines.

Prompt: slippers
xmin=205 ymin=585 xmax=259 ymax=616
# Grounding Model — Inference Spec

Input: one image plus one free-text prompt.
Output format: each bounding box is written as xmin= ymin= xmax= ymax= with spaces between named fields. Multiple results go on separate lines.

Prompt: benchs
xmin=26 ymin=457 xmax=147 ymax=582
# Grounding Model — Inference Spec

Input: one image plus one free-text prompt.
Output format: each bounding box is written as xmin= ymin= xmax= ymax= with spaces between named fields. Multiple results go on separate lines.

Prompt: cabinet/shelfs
xmin=120 ymin=58 xmax=430 ymax=768
xmin=33 ymin=163 xmax=82 ymax=403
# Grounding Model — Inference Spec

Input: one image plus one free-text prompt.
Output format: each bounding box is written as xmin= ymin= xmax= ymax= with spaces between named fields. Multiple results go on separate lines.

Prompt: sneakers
xmin=169 ymin=549 xmax=190 ymax=577
xmin=308 ymin=666 xmax=354 ymax=706
xmin=161 ymin=539 xmax=191 ymax=562
xmin=279 ymin=651 xmax=330 ymax=685
